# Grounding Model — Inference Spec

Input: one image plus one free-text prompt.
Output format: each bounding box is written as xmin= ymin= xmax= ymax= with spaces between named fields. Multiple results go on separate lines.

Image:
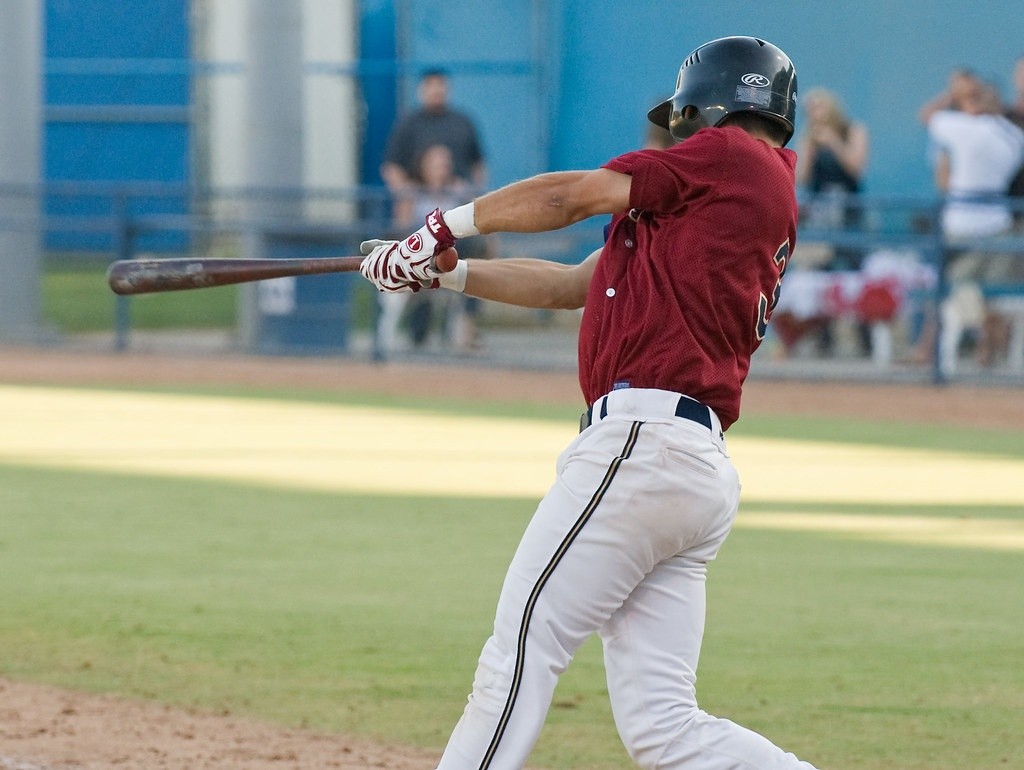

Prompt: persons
xmin=358 ymin=36 xmax=819 ymax=770
xmin=378 ymin=63 xmax=495 ymax=356
xmin=796 ymin=58 xmax=1022 ymax=389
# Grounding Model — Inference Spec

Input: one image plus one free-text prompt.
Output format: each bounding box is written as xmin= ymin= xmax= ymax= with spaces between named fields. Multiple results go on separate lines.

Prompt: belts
xmin=587 ymin=395 xmax=714 ymax=432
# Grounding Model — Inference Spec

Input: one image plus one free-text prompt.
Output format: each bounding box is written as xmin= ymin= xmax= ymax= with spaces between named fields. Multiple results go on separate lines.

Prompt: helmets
xmin=646 ymin=36 xmax=798 ymax=150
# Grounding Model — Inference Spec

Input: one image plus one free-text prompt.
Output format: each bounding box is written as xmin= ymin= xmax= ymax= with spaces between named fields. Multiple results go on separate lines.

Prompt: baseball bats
xmin=108 ymin=244 xmax=459 ymax=297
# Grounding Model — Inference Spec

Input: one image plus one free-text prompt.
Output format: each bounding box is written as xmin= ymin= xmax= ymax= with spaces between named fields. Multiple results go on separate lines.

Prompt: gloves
xmin=358 ymin=206 xmax=458 ymax=293
